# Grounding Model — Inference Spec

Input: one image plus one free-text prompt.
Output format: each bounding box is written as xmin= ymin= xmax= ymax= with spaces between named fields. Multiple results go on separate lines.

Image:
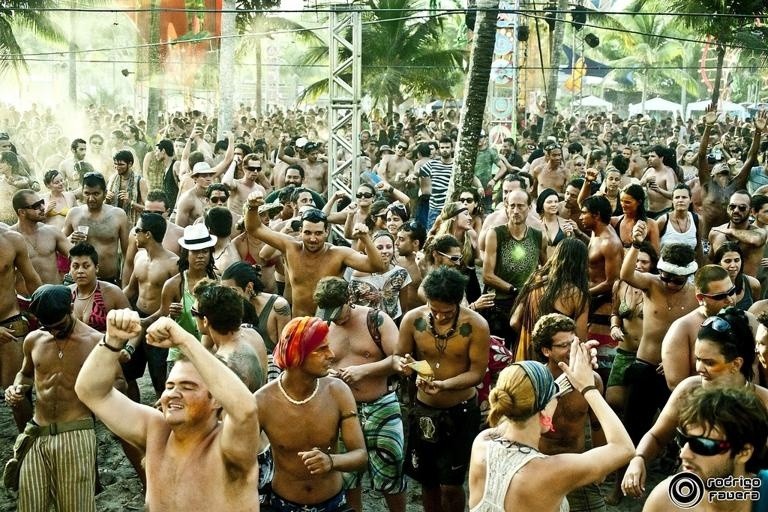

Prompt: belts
xmin=22 ymin=420 xmax=95 ymax=436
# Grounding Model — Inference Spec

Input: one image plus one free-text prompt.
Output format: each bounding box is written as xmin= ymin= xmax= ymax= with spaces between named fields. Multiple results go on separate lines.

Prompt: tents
xmin=571 ymin=95 xmax=611 ymax=111
xmin=690 ymin=99 xmax=740 ymax=108
xmin=629 ymin=97 xmax=682 ymax=112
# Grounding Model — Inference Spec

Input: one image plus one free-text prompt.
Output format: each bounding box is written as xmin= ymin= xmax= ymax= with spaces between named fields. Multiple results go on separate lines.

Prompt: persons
xmin=232 ymin=102 xmax=329 ymax=143
xmin=0 ymin=222 xmax=46 ymax=433
xmin=556 ymin=179 xmax=593 ymax=243
xmin=3 ymin=283 xmax=127 ymax=512
xmin=630 ymin=138 xmax=647 ymax=170
xmin=0 ymin=133 xmax=40 ymax=192
xmin=194 ymin=127 xmax=213 ymax=164
xmin=658 ymin=184 xmax=706 ymax=268
xmin=482 ymin=189 xmax=549 ymax=354
xmin=697 ymin=104 xmax=768 ymax=257
xmin=418 ymin=234 xmax=496 ymax=309
xmin=35 ymin=127 xmax=69 ymax=170
xmin=314 ymin=275 xmax=407 ymax=512
xmin=518 ymin=147 xmax=544 ymax=172
xmin=600 ymin=155 xmax=640 ymax=195
xmin=613 ymin=185 xmax=660 ymax=256
xmin=0 ymin=102 xmax=63 ymax=133
xmin=59 ymin=139 xmax=87 ymax=190
xmin=225 ymin=154 xmax=267 ymax=216
xmin=251 ymin=315 xmax=367 ymax=512
xmin=605 ymin=222 xmax=701 ymax=506
xmin=121 ymin=188 xmax=184 ymax=286
xmin=609 ymin=138 xmax=622 ymax=159
xmin=266 ymin=164 xmax=326 ymax=211
xmin=159 ymin=110 xmax=204 ymax=138
xmin=317 ymin=142 xmax=347 ymax=165
xmin=338 ymin=107 xmax=463 ymax=131
xmin=110 ymin=130 xmax=143 ymax=175
xmin=714 ymin=243 xmax=760 ymax=312
xmin=86 ymin=135 xmax=106 ymax=167
xmin=377 ymin=123 xmax=463 ymax=144
xmin=64 ymin=243 xmax=147 ymax=491
xmin=127 ymin=214 xmax=182 ymax=405
xmin=469 ymin=338 xmax=634 ymax=512
xmin=478 ymin=174 xmax=549 ymax=260
xmin=74 ymin=308 xmax=260 ymax=511
xmin=203 ymin=261 xmax=291 ymax=385
xmin=752 ymin=195 xmax=768 ymax=228
xmin=141 ymin=224 xmax=222 ymax=380
xmin=175 ymin=163 xmax=216 ymax=225
xmin=538 ymin=188 xmax=574 ymax=259
xmin=379 ymin=140 xmax=415 ymax=182
xmin=393 ymin=268 xmax=490 ymax=511
xmin=474 ymin=129 xmax=507 ymax=215
xmin=662 ymin=264 xmax=759 ymax=392
xmin=169 ymin=125 xmax=234 ymax=224
xmin=706 ymin=115 xmax=768 ymax=178
xmin=278 ymin=134 xmax=328 ymax=199
xmin=682 ymin=151 xmax=698 ymax=181
xmin=214 ymin=140 xmax=227 ymax=160
xmin=643 ymin=387 xmax=768 ymax=512
xmin=63 ymin=171 xmax=129 ymax=288
xmin=728 ymin=139 xmax=768 ymax=194
xmin=257 ymin=188 xmax=313 ymax=298
xmin=0 ymin=152 xmax=29 ymax=227
xmin=202 ymin=208 xmax=242 ymax=274
xmin=622 ymin=149 xmax=641 ymax=179
xmin=582 ymin=197 xmax=622 ymax=311
xmin=194 ymin=184 xmax=245 ymax=238
xmin=123 ymin=124 xmax=152 ymax=170
xmin=105 ymin=149 xmax=149 ymax=226
xmin=404 ymin=137 xmax=484 ymax=236
xmin=425 ymin=201 xmax=480 ymax=305
xmin=516 ymin=171 xmax=534 ymax=193
xmin=640 ymin=146 xmax=676 ymax=219
xmin=155 ymin=138 xmax=181 ymax=216
xmin=243 ymin=193 xmax=386 ymax=316
xmin=69 ymin=162 xmax=93 ymax=202
xmin=79 ymin=102 xmax=146 ymax=134
xmin=530 ymin=311 xmax=606 ymax=512
xmin=499 ymin=137 xmax=523 ymax=175
xmin=320 ymin=184 xmax=410 ymax=229
xmin=234 ymin=143 xmax=273 ymax=195
xmin=230 ymin=190 xmax=285 ymax=294
xmin=385 ymin=201 xmax=409 ymax=233
xmin=707 ymin=191 xmax=767 ymax=278
xmin=533 ymin=142 xmax=571 ymax=201
xmin=42 ymin=171 xmax=78 ymax=276
xmin=7 ymin=190 xmax=79 ymax=329
xmin=620 ymin=307 xmax=768 ymax=499
xmin=350 ymin=200 xmax=389 ymax=253
xmin=755 ymin=313 xmax=768 ymax=368
xmin=747 ymin=298 xmax=768 ymax=317
xmin=372 ymin=145 xmax=395 ymax=174
xmin=393 ymin=221 xmax=426 ymax=314
xmin=675 ymin=142 xmax=700 ymax=152
xmin=515 ymin=110 xmax=706 ymax=142
xmin=253 ymin=146 xmax=271 ymax=174
xmin=599 ymin=168 xmax=625 ymax=217
xmin=357 ymin=129 xmax=378 ymax=156
xmin=529 ymin=139 xmax=555 ymax=174
xmin=268 ymin=146 xmax=296 ymax=190
xmin=603 ymin=243 xmax=660 ymax=412
xmin=460 ymin=188 xmax=482 ymax=236
xmin=510 ymin=235 xmax=589 ymax=364
xmin=187 ymin=279 xmax=273 ymax=511
xmin=566 ymin=143 xmax=607 ymax=180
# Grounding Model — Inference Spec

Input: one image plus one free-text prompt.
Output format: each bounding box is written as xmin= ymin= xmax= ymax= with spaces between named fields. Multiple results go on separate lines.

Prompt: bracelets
xmin=634 ymin=453 xmax=646 ymax=460
xmin=247 ymin=203 xmax=258 ymax=210
xmin=579 ymin=384 xmax=598 ymax=396
xmin=325 ymin=452 xmax=335 ymax=472
xmin=97 ymin=333 xmax=128 ymax=353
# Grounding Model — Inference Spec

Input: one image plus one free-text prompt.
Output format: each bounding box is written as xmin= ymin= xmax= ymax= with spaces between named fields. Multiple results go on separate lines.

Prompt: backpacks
xmin=347 ymin=230 xmax=410 ymax=330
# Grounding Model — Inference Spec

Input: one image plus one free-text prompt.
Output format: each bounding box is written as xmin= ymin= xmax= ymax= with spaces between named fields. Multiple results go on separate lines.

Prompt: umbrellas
xmin=426 ymin=101 xmax=458 ymax=107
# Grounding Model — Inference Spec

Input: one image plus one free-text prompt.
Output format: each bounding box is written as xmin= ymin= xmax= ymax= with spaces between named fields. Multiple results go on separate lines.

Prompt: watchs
xmin=609 ymin=322 xmax=620 ymax=330
xmin=631 ymin=239 xmax=642 ymax=249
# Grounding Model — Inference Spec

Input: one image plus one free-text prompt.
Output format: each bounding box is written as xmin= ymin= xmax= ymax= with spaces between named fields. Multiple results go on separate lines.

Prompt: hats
xmin=296 ymin=138 xmax=308 ymax=148
xmin=29 ymin=283 xmax=72 ymax=323
xmin=236 ymin=200 xmax=284 ymax=230
xmin=177 ymin=224 xmax=218 ymax=251
xmin=314 ymin=276 xmax=350 ymax=319
xmin=190 ymin=163 xmax=216 ymax=177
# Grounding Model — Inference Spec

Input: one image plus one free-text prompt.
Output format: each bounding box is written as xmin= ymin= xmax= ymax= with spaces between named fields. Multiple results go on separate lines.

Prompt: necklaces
xmin=215 ymin=243 xmax=229 ymax=260
xmin=429 ymin=308 xmax=461 ymax=338
xmin=50 ymin=319 xmax=74 ymax=358
xmin=664 ymin=291 xmax=685 ymax=311
xmin=725 ymin=222 xmax=750 ymax=244
xmin=74 ymin=282 xmax=99 ymax=312
xmin=14 ymin=222 xmax=38 ymax=250
xmin=278 ymin=371 xmax=321 ymax=404
xmin=676 ymin=217 xmax=689 ymax=234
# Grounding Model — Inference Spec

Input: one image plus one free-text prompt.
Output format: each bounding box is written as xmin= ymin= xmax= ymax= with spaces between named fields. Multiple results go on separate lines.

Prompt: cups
xmin=77 ymin=226 xmax=89 ymax=241
xmin=487 ymin=288 xmax=496 ymax=297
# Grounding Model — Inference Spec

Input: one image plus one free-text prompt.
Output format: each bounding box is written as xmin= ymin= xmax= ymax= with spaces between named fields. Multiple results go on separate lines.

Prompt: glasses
xmin=408 ymin=221 xmax=420 ymax=241
xmin=660 ymin=275 xmax=687 ymax=286
xmin=700 ymin=316 xmax=738 ymax=346
xmin=306 ymin=144 xmax=318 ymax=150
xmin=22 ymin=199 xmax=45 ymax=210
xmin=388 ymin=204 xmax=409 ymax=217
xmin=548 ymin=145 xmax=562 ymax=151
xmin=728 ymin=203 xmax=747 ymax=214
xmin=374 ymin=214 xmax=388 ymax=221
xmin=135 ymin=227 xmax=150 ymax=233
xmin=676 ymin=428 xmax=731 ymax=457
xmin=398 ymin=145 xmax=408 ymax=152
xmin=301 ymin=210 xmax=326 ymax=220
xmin=41 ymin=319 xmax=68 ymax=331
xmin=548 ymin=340 xmax=574 ymax=348
xmin=459 ymin=197 xmax=474 ymax=203
xmin=82 ymin=171 xmax=103 ymax=180
xmin=438 ymin=251 xmax=462 ymax=261
xmin=190 ymin=307 xmax=206 ymax=318
xmin=143 ymin=210 xmax=164 ymax=217
xmin=246 ymin=167 xmax=261 ymax=172
xmin=211 ymin=195 xmax=226 ymax=203
xmin=702 ymin=286 xmax=737 ymax=301
xmin=356 ymin=192 xmax=374 ymax=198
